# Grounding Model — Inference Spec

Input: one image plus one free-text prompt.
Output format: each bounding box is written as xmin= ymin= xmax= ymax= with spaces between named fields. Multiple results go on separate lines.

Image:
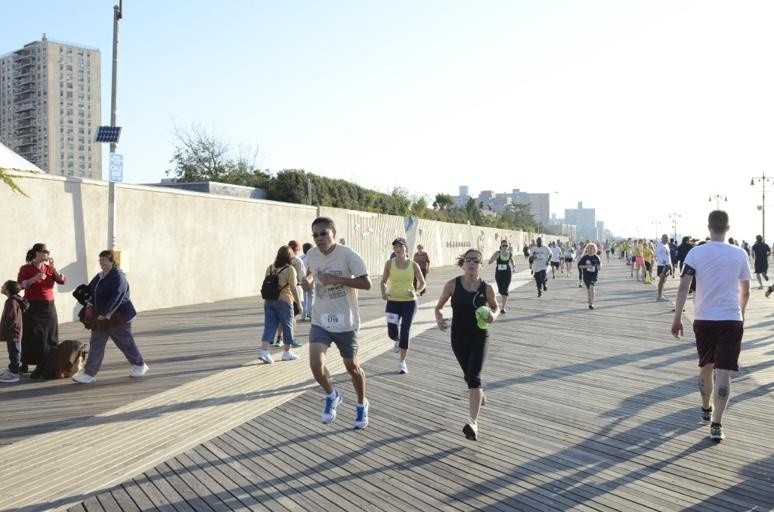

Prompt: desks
xmin=747 ymin=170 xmax=774 ymax=247
xmin=708 ymin=193 xmax=729 ymax=215
xmin=647 ymin=215 xmax=664 ymax=242
xmin=667 ymin=211 xmax=684 ymax=244
xmin=520 ymin=190 xmax=562 ymax=232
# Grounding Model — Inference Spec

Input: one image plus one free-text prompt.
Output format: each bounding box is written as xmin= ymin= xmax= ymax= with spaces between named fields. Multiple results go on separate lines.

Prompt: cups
xmin=464 ymin=256 xmax=481 ymax=264
xmin=312 ymin=228 xmax=333 ymax=238
xmin=37 ymin=249 xmax=52 ymax=254
xmin=502 ymin=244 xmax=509 ymax=247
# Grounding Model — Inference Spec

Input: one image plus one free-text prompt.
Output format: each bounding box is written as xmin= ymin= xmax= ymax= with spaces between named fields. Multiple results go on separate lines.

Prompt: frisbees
xmin=463 ymin=264 xmax=464 ymax=267
xmin=480 ymin=264 xmax=481 ymax=267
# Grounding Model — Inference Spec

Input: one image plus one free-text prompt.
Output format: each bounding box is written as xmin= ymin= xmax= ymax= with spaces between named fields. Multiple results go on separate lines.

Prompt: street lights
xmin=72 ymin=284 xmax=90 ymax=305
xmin=43 ymin=340 xmax=88 ymax=381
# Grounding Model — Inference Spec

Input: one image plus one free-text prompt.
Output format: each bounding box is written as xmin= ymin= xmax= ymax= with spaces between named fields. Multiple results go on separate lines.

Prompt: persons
xmin=625 ymin=237 xmax=659 ymax=284
xmin=488 ymin=240 xmax=516 ymax=315
xmin=261 ymin=245 xmax=304 ymax=363
xmin=577 ymin=243 xmax=602 ymax=309
xmin=548 ymin=240 xmax=577 ymax=280
xmin=301 ymin=217 xmax=372 ymax=429
xmin=413 ymin=243 xmax=431 ymax=296
xmin=529 ymin=237 xmax=553 ymax=298
xmin=665 ymin=238 xmax=679 ymax=278
xmin=435 ymin=249 xmax=500 ymax=440
xmin=300 ymin=243 xmax=314 ymax=321
xmin=523 ymin=243 xmax=530 ymax=260
xmin=603 ymin=239 xmax=627 ymax=264
xmin=729 ymin=237 xmax=751 ymax=264
xmin=574 ymin=240 xmax=586 ymax=287
xmin=671 ymin=211 xmax=753 ymax=439
xmin=751 ymin=235 xmax=771 ymax=289
xmin=271 ymin=240 xmax=307 ymax=348
xmin=583 ymin=240 xmax=605 ymax=258
xmin=765 ymin=286 xmax=773 ymax=297
xmin=381 ymin=237 xmax=426 ymax=375
xmin=71 ymin=251 xmax=149 ymax=384
xmin=527 ymin=239 xmax=537 ymax=275
xmin=390 ymin=245 xmax=409 ymax=259
xmin=18 ymin=243 xmax=66 ymax=375
xmin=655 ymin=234 xmax=673 ymax=302
xmin=675 ymin=236 xmax=711 ymax=299
xmin=0 ymin=280 xmax=30 ymax=383
xmin=507 ymin=242 xmax=513 ymax=252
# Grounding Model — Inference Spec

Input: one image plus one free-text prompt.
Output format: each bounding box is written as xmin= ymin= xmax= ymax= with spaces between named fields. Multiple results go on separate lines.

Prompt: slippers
xmin=260 ymin=264 xmax=291 ymax=300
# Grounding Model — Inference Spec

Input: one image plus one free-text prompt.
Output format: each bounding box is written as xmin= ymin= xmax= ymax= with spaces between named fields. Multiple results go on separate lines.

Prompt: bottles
xmin=392 ymin=237 xmax=406 ymax=245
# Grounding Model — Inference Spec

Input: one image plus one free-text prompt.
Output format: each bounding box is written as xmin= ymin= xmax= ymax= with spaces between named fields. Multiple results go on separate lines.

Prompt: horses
xmin=679 ymin=263 xmax=686 ymax=276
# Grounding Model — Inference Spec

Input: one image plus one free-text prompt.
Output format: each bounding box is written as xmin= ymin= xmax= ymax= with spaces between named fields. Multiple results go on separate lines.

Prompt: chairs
xmin=0 ymin=368 xmax=22 ymax=383
xmin=709 ymin=423 xmax=725 ymax=441
xmin=462 ymin=419 xmax=479 ymax=441
xmin=398 ymin=360 xmax=408 ymax=375
xmin=129 ymin=363 xmax=150 ymax=378
xmin=655 ymin=295 xmax=669 ymax=302
xmin=320 ymin=386 xmax=343 ymax=425
xmin=700 ymin=406 xmax=713 ymax=425
xmin=393 ymin=340 xmax=400 ymax=352
xmin=71 ymin=373 xmax=97 ymax=384
xmin=481 ymin=396 xmax=487 ymax=406
xmin=258 ymin=350 xmax=275 ymax=365
xmin=354 ymin=396 xmax=371 ymax=429
xmin=281 ymin=351 xmax=299 ymax=361
